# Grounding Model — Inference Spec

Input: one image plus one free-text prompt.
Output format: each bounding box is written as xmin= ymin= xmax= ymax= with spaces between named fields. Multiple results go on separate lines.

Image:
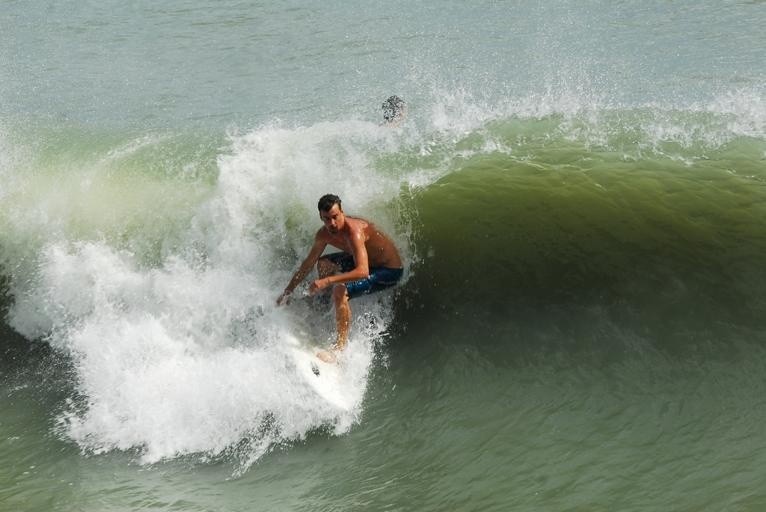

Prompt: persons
xmin=380 ymin=96 xmax=409 ymax=125
xmin=276 ymin=193 xmax=403 ymax=364
